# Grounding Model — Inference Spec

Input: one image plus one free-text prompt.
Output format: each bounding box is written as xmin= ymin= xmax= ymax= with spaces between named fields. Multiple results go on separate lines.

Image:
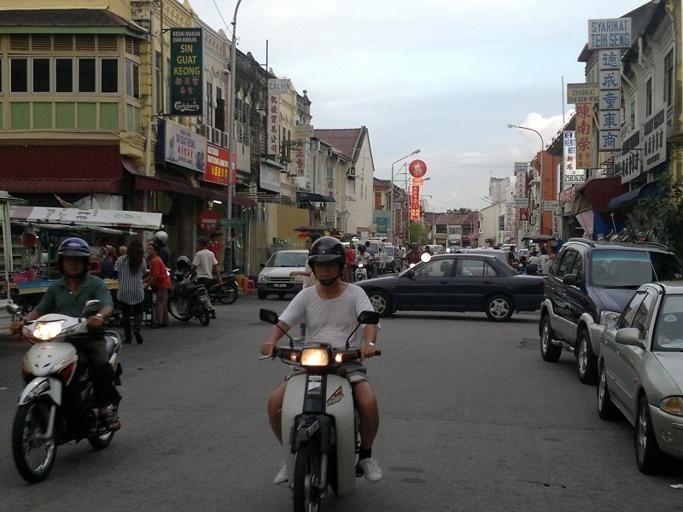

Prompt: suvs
xmin=534 ymin=236 xmax=682 ymax=385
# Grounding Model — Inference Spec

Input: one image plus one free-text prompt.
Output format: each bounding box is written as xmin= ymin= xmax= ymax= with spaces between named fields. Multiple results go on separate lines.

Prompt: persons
xmin=260 ymin=235 xmax=384 ymax=485
xmin=345 ymin=242 xmax=432 ymax=283
xmin=104 ymin=231 xmax=173 ymax=344
xmin=440 ymin=261 xmax=449 ymax=277
xmin=508 ymin=241 xmax=558 ymax=275
xmin=190 ymin=235 xmax=222 ymax=319
xmin=12 ymin=236 xmax=121 ymax=433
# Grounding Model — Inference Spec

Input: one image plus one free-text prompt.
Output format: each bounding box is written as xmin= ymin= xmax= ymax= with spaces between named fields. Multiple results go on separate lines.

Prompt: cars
xmin=336 ymin=237 xmax=562 ymax=271
xmin=590 ymin=277 xmax=682 ymax=478
xmin=348 ymin=251 xmax=546 ymax=323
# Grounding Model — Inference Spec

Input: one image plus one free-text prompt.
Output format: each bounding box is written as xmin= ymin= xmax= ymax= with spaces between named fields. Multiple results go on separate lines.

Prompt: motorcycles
xmin=3 ymin=298 xmax=126 ymax=486
xmin=167 ymin=256 xmax=217 ymax=327
xmin=348 ymin=259 xmax=374 ymax=282
xmin=185 ymin=267 xmax=242 ymax=306
xmin=254 ymin=304 xmax=382 ymax=511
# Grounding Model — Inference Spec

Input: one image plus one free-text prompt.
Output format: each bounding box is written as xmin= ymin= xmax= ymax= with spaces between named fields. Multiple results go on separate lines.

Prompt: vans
xmin=254 ymin=248 xmax=310 ymax=300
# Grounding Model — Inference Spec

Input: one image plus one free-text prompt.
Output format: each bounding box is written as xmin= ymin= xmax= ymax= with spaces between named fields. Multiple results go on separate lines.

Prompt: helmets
xmin=308 ymin=236 xmax=346 ymax=266
xmin=57 ymin=238 xmax=92 ymax=261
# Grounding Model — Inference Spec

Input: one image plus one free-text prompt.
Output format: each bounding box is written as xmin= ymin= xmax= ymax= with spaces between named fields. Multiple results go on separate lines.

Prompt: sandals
xmin=99 ymin=409 xmax=120 ymax=431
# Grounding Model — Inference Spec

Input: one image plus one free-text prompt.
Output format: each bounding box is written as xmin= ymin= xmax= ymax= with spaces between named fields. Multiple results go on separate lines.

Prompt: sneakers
xmin=359 ymin=458 xmax=383 ymax=482
xmin=273 ymin=464 xmax=288 ymax=484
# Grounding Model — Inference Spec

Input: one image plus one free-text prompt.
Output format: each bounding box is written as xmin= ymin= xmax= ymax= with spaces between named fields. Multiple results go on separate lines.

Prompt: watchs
xmin=366 ymin=342 xmax=375 ymax=346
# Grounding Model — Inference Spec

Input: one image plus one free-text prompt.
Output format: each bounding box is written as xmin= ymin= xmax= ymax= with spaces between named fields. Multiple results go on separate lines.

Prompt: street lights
xmin=420 ymin=193 xmax=432 ymax=198
xmin=506 ymin=122 xmax=544 ymax=236
xmin=388 ymin=149 xmax=422 ymax=242
xmin=483 ymin=195 xmax=500 ymax=204
xmin=421 ymin=176 xmax=433 ymax=184
